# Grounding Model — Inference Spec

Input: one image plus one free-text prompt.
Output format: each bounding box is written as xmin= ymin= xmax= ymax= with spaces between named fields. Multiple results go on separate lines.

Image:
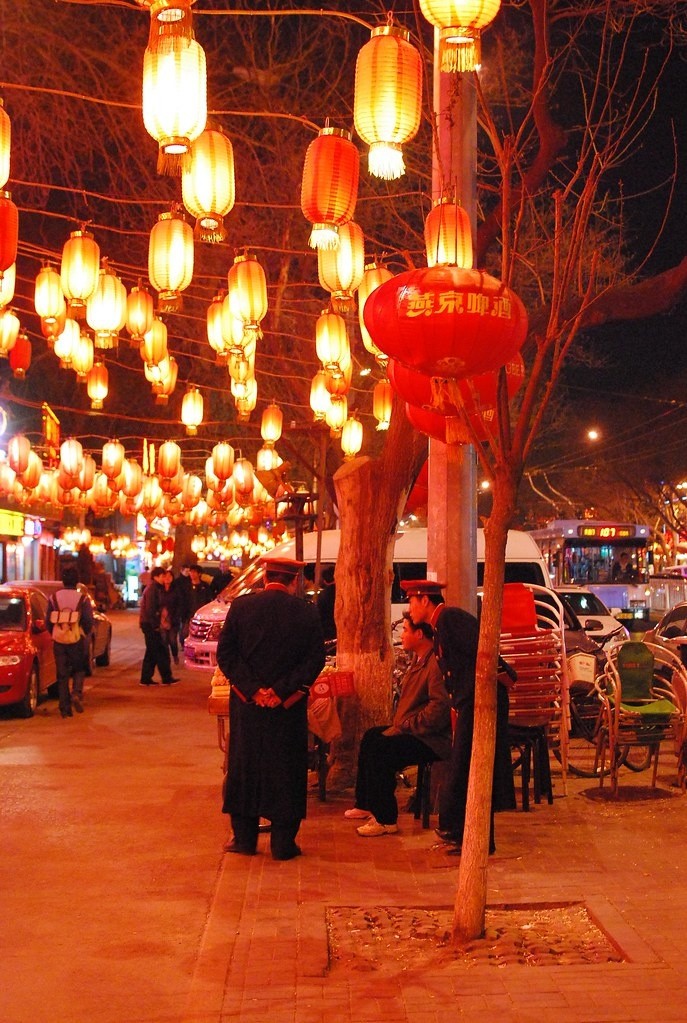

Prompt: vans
xmin=182 ymin=525 xmax=555 ymax=672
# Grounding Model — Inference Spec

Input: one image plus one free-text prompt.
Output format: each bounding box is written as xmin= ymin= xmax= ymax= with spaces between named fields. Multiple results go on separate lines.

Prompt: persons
xmin=569 ymin=551 xmax=639 ymax=584
xmin=138 ymin=560 xmax=235 ymax=686
xmin=400 ymin=580 xmax=516 ymax=856
xmin=345 ymin=611 xmax=452 ymax=836
xmin=216 ymin=556 xmax=327 ymax=861
xmin=317 ymin=565 xmax=337 ymax=639
xmin=45 ymin=562 xmax=94 ymax=718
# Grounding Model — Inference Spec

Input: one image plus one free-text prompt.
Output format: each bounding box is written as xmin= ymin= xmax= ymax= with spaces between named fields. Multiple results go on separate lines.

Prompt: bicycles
xmin=324 ymin=614 xmax=419 ymax=789
xmin=551 ymin=625 xmax=654 ymax=779
xmin=307 ymin=639 xmax=338 ymax=802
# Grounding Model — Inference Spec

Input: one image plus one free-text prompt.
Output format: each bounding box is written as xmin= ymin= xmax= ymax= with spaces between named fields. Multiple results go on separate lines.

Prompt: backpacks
xmin=49 ymin=589 xmax=87 ymax=645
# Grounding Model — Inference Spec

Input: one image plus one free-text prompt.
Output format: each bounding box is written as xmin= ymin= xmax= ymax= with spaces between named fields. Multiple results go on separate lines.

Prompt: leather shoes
xmin=434 ymin=828 xmax=456 ymax=841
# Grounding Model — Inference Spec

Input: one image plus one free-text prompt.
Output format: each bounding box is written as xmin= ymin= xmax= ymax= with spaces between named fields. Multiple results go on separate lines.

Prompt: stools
xmin=415 ymin=752 xmax=447 ymax=829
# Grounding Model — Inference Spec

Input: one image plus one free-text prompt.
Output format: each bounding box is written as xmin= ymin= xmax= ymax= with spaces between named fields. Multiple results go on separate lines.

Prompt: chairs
xmin=476 ymin=582 xmax=572 ymax=811
xmin=593 ymin=640 xmax=687 ymax=802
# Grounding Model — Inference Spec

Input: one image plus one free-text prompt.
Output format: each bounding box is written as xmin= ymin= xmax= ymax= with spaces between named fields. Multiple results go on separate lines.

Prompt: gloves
xmin=381 ymin=726 xmax=404 ymax=737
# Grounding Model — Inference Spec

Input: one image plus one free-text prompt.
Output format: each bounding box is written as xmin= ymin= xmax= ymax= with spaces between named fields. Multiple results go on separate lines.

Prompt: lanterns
xmin=0 ymin=0 xmax=524 ymax=560
xmin=362 ymin=264 xmax=527 ymax=527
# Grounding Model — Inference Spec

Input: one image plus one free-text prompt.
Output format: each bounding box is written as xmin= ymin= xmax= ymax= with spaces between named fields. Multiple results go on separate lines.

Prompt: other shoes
xmin=70 ymin=695 xmax=84 ymax=713
xmin=58 ymin=702 xmax=73 ymax=717
xmin=173 ymin=655 xmax=179 ymax=665
xmin=180 ymin=642 xmax=185 ymax=651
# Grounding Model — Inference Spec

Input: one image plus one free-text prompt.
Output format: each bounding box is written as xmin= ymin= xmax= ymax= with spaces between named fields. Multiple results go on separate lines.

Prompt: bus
xmin=528 ymin=519 xmax=656 ymax=631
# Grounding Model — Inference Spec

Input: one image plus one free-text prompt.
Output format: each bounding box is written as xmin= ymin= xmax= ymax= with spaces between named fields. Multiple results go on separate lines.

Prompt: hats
xmin=263 ymin=557 xmax=307 ymax=576
xmin=399 ymin=579 xmax=446 ymax=595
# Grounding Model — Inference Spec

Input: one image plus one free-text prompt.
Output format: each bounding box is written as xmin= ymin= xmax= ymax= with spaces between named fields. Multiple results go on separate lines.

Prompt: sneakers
xmin=272 ymin=841 xmax=302 ymax=861
xmin=161 ymin=678 xmax=181 ymax=687
xmin=356 ymin=815 xmax=398 ymax=837
xmin=139 ymin=677 xmax=160 ymax=686
xmin=221 ymin=837 xmax=257 ymax=855
xmin=345 ymin=805 xmax=372 ymax=819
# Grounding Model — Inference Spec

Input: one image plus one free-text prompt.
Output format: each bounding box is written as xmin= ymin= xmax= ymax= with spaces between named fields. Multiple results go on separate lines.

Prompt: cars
xmin=553 ymin=584 xmax=630 ymax=661
xmin=532 ymin=585 xmax=609 ymax=695
xmin=3 ymin=580 xmax=113 ymax=679
xmin=0 ymin=584 xmax=59 ymax=720
xmin=639 ymin=600 xmax=687 ymax=672
